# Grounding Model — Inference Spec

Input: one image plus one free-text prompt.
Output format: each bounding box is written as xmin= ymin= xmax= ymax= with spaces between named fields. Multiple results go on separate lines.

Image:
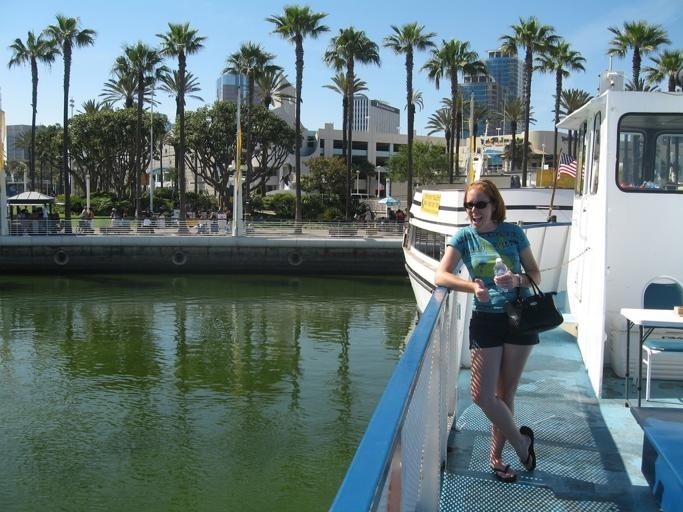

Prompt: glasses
xmin=464 ymin=200 xmax=492 ymax=209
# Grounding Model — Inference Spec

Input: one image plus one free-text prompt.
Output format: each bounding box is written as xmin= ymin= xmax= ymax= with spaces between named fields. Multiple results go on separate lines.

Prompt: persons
xmin=435 ymin=180 xmax=541 ymax=481
xmin=354 ymin=207 xmax=407 ymax=232
xmin=17 ymin=202 xmax=232 ymax=236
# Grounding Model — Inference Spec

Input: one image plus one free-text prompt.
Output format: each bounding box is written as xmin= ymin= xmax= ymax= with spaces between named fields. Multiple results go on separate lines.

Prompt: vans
xmin=351 ymin=193 xmax=376 ymax=204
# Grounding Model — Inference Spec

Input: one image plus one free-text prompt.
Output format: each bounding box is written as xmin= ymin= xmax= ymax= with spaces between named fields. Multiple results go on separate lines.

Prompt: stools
xmin=642 ymin=338 xmax=683 ymax=401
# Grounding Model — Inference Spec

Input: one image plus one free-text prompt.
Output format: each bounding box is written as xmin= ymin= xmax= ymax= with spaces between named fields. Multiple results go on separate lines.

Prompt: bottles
xmin=492 ymin=258 xmax=511 ymax=293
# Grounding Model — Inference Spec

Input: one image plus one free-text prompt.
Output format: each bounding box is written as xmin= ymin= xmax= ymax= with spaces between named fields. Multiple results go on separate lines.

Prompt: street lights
xmin=376 ymin=165 xmax=380 ymax=197
xmin=83 ymin=173 xmax=90 ymax=211
xmin=356 ymin=170 xmax=360 ymax=194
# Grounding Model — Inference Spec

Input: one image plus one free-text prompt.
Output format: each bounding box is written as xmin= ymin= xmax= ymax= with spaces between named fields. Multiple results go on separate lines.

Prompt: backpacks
xmin=503 ymin=273 xmax=564 ymax=337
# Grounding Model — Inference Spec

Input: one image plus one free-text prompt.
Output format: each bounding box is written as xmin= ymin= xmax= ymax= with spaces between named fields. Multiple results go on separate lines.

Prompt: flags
xmin=557 ymin=152 xmax=584 ymax=181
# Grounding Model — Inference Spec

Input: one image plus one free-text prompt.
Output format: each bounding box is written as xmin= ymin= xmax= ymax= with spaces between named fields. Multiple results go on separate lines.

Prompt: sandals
xmin=520 ymin=426 xmax=536 ymax=471
xmin=491 ymin=464 xmax=517 ymax=483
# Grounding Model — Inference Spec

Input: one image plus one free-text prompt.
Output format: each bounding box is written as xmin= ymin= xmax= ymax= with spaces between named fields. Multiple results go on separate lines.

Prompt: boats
xmin=399 ymin=53 xmax=596 ymax=319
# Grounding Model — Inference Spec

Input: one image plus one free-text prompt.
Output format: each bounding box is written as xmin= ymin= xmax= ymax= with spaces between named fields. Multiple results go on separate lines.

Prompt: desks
xmin=619 ymin=308 xmax=683 ymax=410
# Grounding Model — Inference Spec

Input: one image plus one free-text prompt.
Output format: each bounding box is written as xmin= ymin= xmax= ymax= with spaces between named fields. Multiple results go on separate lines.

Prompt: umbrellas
xmin=378 ymin=196 xmax=401 ymax=205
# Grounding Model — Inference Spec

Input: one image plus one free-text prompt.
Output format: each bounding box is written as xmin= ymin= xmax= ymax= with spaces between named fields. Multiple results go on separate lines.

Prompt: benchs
xmin=365 ymin=227 xmax=378 ymax=236
xmin=328 ymin=228 xmax=358 ymax=237
xmin=136 ymin=227 xmax=149 ymax=233
xmin=99 ymin=226 xmax=133 ymax=234
